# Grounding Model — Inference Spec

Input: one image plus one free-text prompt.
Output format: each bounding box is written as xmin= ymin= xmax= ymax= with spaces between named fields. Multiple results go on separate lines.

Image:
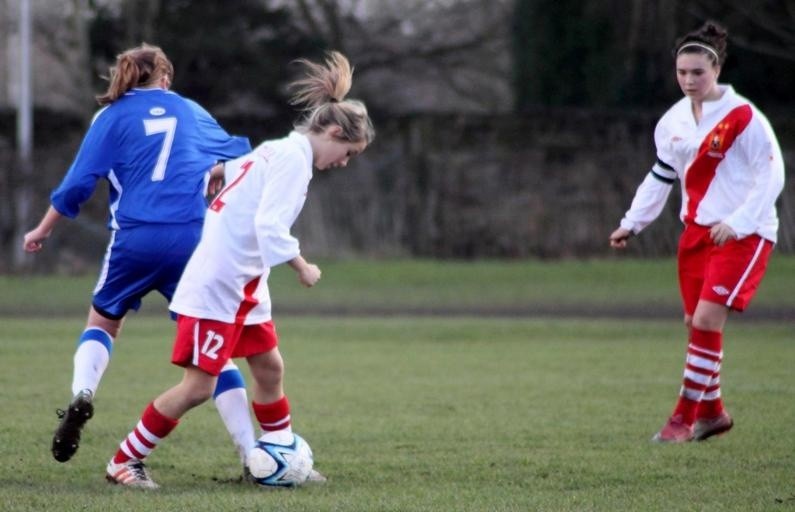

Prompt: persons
xmin=609 ymin=21 xmax=786 ymax=443
xmin=103 ymin=49 xmax=376 ymax=491
xmin=20 ymin=40 xmax=255 ymax=480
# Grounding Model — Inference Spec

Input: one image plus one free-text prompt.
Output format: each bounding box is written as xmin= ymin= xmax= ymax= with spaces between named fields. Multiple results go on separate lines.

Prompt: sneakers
xmin=650 ymin=409 xmax=734 ymax=441
xmin=105 ymin=456 xmax=162 ymax=490
xmin=53 ymin=389 xmax=94 ymax=462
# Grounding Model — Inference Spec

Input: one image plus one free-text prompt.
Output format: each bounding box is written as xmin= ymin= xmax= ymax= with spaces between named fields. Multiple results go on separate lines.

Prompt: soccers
xmin=247 ymin=429 xmax=314 ymax=485
